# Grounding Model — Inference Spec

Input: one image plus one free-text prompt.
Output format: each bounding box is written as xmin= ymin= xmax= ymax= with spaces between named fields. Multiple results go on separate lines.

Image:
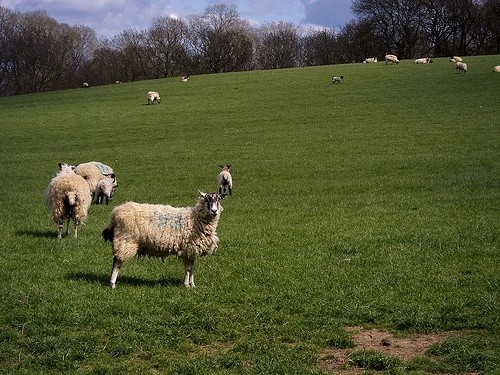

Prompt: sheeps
xmin=385 ymin=54 xmax=399 ymax=65
xmin=450 ymin=55 xmax=467 ymax=74
xmin=181 ymin=76 xmax=190 ymax=82
xmin=362 ymin=57 xmax=379 ymax=64
xmin=332 ymin=75 xmax=344 ymax=84
xmin=147 ymin=91 xmax=160 ymax=105
xmin=218 ymin=164 xmax=234 ymax=196
xmin=493 ymin=64 xmax=500 ymax=72
xmin=415 ymin=57 xmax=434 ymax=64
xmin=102 ymin=190 xmax=224 ymax=288
xmin=44 ymin=161 xmax=118 ymax=240
xmin=114 ymin=81 xmax=121 ymax=84
xmin=82 ymin=83 xmax=89 ymax=88
